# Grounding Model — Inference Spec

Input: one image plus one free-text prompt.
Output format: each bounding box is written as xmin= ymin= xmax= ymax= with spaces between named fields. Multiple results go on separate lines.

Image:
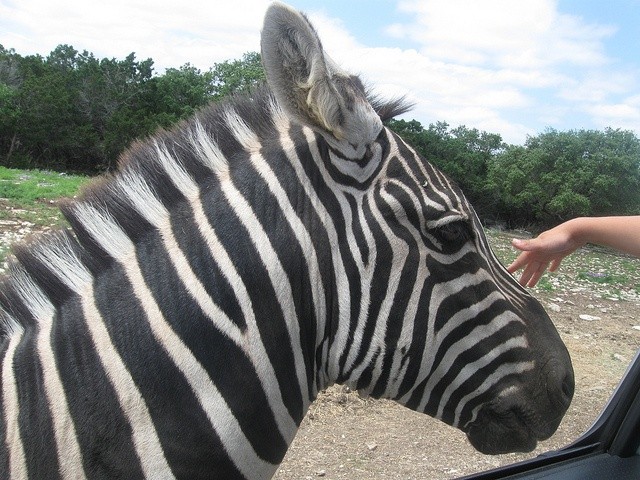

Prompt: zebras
xmin=0 ymin=3 xmax=575 ymax=480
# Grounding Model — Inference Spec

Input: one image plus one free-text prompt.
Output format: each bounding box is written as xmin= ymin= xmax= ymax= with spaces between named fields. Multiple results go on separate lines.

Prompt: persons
xmin=507 ymin=216 xmax=640 ymax=286
